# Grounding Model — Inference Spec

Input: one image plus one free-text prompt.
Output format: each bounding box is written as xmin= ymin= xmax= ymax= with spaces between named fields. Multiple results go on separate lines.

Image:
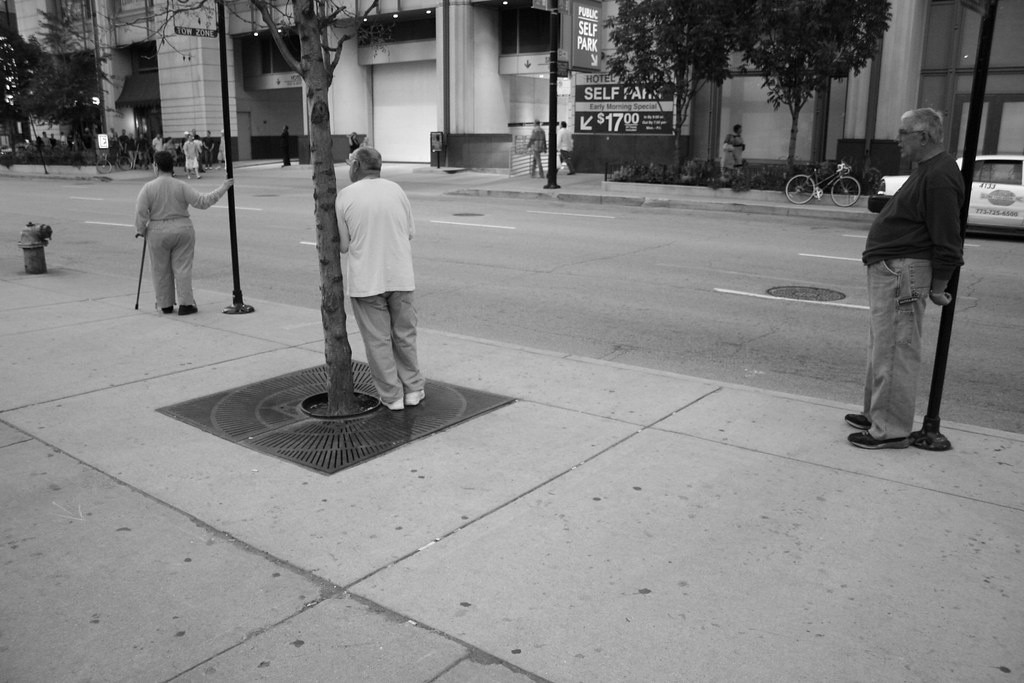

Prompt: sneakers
xmin=845 ymin=414 xmax=872 ymax=429
xmin=405 ymin=390 xmax=425 ymax=406
xmin=848 ymin=430 xmax=910 ymax=449
xmin=380 ymin=397 xmax=404 ymax=410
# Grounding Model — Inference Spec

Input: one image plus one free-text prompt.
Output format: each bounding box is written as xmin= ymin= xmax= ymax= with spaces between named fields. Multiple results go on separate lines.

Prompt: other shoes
xmin=178 ymin=305 xmax=197 ymax=315
xmin=567 ymin=170 xmax=576 ymax=175
xmin=161 ymin=306 xmax=173 ymax=313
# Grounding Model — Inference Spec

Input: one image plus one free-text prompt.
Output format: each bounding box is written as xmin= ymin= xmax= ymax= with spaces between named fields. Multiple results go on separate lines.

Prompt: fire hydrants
xmin=17 ymin=220 xmax=54 ymax=274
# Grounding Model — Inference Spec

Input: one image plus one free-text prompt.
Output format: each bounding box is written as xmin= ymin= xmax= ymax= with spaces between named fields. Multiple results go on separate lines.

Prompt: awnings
xmin=116 ymin=73 xmax=159 ymax=108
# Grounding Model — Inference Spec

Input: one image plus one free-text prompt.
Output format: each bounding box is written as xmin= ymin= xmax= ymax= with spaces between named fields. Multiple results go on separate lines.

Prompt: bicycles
xmin=96 ymin=147 xmax=136 ymax=175
xmin=783 ymin=157 xmax=864 ymax=207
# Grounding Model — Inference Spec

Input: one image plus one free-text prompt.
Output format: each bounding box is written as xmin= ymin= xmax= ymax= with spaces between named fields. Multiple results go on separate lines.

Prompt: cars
xmin=868 ymin=153 xmax=1023 ymax=235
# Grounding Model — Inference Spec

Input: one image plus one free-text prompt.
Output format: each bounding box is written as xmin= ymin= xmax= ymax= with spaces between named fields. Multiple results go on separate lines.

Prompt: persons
xmin=36 ymin=128 xmax=152 ymax=170
xmin=845 ymin=108 xmax=963 ymax=450
xmin=280 ymin=125 xmax=291 ymax=166
xmin=203 ymin=131 xmax=215 ymax=169
xmin=215 ymin=130 xmax=226 ymax=170
xmin=136 ymin=151 xmax=233 ymax=314
xmin=336 ymin=146 xmax=425 ymax=410
xmin=557 ymin=121 xmax=576 ymax=174
xmin=720 ymin=125 xmax=745 ymax=182
xmin=527 ymin=121 xmax=547 ymax=177
xmin=179 ymin=129 xmax=206 ymax=178
xmin=152 ymin=133 xmax=178 ymax=176
xmin=348 ymin=132 xmax=360 ymax=159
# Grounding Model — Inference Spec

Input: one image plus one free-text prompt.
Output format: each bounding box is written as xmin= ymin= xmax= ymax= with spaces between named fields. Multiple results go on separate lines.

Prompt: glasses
xmin=898 ymin=129 xmax=932 ymax=139
